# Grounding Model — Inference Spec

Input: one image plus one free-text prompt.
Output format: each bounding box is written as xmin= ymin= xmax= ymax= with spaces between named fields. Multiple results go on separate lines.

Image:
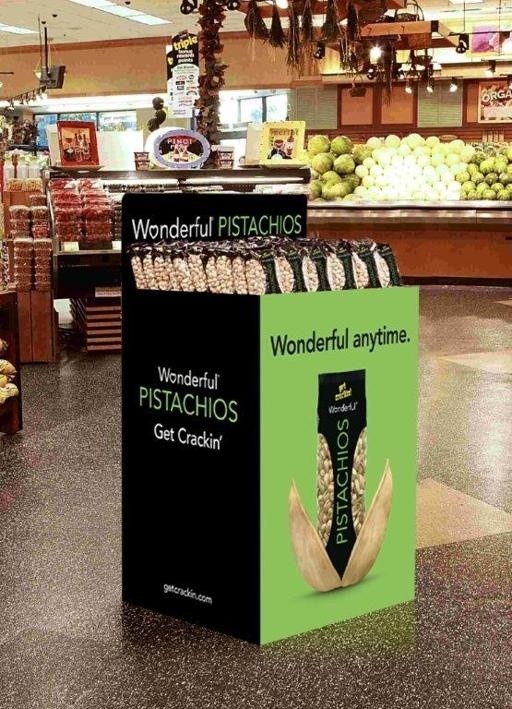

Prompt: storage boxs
xmin=74 ymin=297 xmax=123 ymax=353
xmin=68 ymin=298 xmax=77 ymax=329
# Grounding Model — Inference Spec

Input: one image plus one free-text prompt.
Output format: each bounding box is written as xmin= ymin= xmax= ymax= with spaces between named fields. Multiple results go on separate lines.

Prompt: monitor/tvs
xmin=38 ymin=65 xmax=65 ymax=89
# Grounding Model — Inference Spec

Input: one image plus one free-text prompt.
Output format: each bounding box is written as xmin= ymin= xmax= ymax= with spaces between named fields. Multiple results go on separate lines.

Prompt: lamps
xmin=456 ymin=33 xmax=470 ymax=54
xmin=405 ymin=77 xmax=414 ymax=94
xmin=450 ymin=75 xmax=459 ymax=92
xmin=313 ymin=43 xmax=326 ymax=59
xmin=426 ymin=78 xmax=436 ymax=94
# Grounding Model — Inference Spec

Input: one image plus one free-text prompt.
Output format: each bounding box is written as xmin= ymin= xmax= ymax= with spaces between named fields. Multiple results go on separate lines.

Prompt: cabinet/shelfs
xmin=56 ymin=164 xmax=314 ymax=304
xmin=0 ymin=166 xmax=53 ymax=367
xmin=0 ymin=292 xmax=25 ymax=437
xmin=118 ymin=186 xmax=426 ymax=644
xmin=303 ymin=124 xmax=512 ymax=288
